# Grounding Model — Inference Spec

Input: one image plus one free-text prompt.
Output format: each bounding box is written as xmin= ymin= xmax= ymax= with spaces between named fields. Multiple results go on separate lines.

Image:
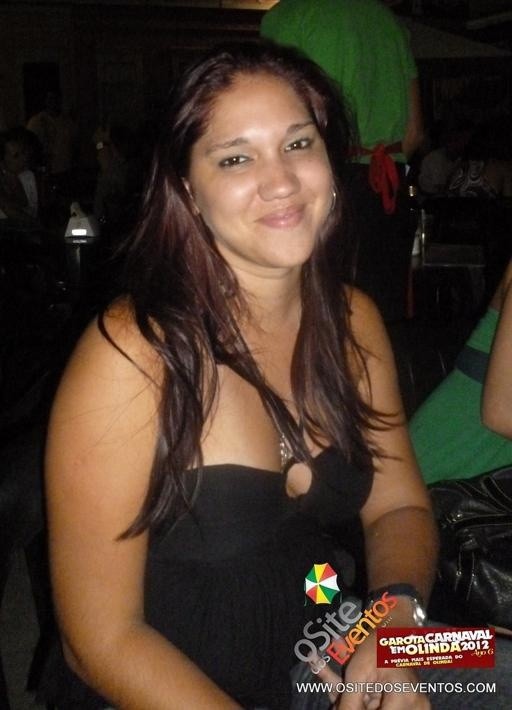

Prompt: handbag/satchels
xmin=429 ymin=460 xmax=512 ymax=633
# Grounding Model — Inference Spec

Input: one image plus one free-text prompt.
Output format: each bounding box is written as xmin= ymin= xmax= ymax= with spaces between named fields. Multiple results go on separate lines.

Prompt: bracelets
xmin=357 ymin=582 xmax=429 ymax=628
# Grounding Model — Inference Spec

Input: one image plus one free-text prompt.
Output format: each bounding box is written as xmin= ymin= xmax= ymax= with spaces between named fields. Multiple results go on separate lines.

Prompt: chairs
xmin=26 ymin=529 xmax=61 ymax=696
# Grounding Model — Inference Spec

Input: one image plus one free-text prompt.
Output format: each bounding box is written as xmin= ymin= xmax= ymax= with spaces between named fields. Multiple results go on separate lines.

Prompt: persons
xmin=256 ymin=1 xmax=427 ymax=423
xmin=409 ymin=261 xmax=511 ymax=487
xmin=24 ymin=37 xmax=446 ymax=710
